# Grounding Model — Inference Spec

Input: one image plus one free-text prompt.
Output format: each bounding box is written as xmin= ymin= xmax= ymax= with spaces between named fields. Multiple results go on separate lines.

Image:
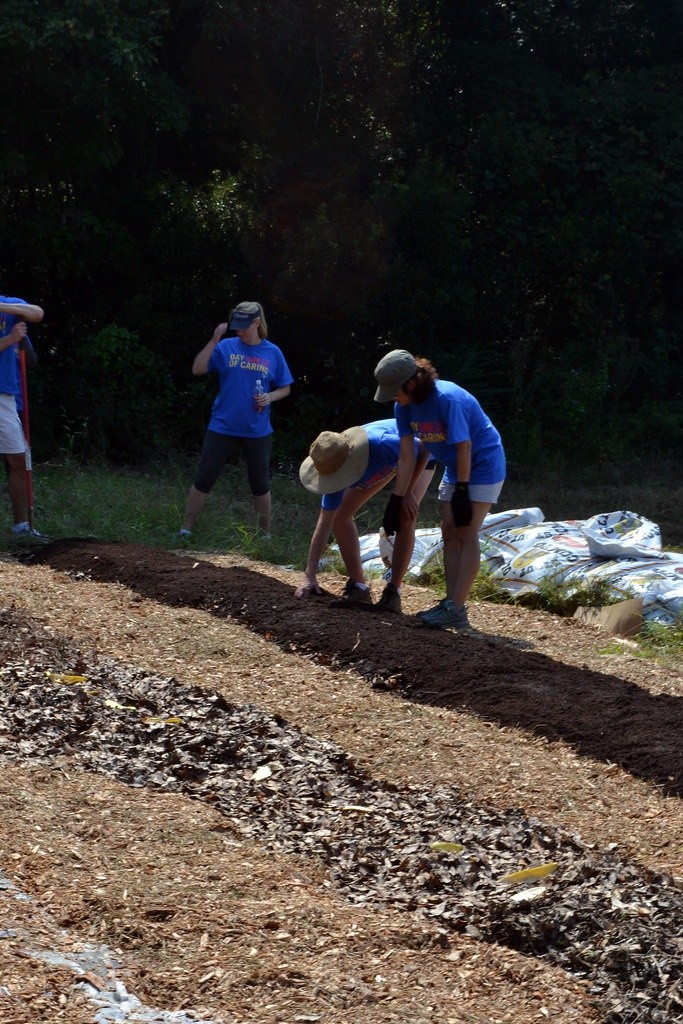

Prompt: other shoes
xmin=377 ymin=586 xmax=402 ymax=612
xmin=338 ymin=585 xmax=373 ymax=603
xmin=11 ymin=526 xmax=45 ymax=541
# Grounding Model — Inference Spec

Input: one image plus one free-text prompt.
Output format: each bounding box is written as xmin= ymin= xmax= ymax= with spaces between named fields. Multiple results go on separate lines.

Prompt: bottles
xmin=254 ymin=379 xmax=265 ymax=413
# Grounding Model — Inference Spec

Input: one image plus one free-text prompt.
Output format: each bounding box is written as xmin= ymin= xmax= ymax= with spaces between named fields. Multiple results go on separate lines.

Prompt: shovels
xmin=18 ymin=338 xmax=40 ymax=535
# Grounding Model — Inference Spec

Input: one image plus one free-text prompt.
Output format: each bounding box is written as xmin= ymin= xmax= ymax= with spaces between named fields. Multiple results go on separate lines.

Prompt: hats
xmin=299 ymin=425 xmax=369 ymax=495
xmin=229 ymin=306 xmax=260 ymax=331
xmin=374 ymin=350 xmax=417 ymax=402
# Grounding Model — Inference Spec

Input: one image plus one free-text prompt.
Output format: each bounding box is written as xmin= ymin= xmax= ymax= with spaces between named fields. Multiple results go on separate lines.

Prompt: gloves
xmin=450 ymin=481 xmax=474 ymax=528
xmin=383 ymin=493 xmax=404 ymax=536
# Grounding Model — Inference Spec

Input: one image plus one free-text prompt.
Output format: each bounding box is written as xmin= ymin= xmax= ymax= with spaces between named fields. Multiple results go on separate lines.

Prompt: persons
xmin=179 ymin=302 xmax=294 ymax=539
xmin=301 ymin=417 xmax=436 ymax=614
xmin=0 ymin=294 xmax=43 ymax=539
xmin=373 ymin=349 xmax=507 ymax=630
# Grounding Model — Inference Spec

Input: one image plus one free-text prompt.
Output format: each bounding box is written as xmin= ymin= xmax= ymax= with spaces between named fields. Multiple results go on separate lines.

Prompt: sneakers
xmin=418 ymin=596 xmax=470 ymax=629
